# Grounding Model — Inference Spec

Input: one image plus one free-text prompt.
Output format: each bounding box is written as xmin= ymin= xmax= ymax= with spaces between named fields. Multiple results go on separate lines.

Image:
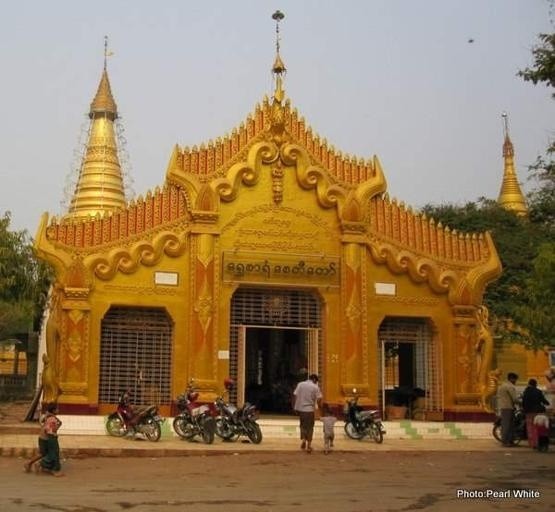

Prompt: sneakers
xmin=23 ymin=460 xmax=40 ymax=473
xmin=301 ymin=442 xmax=312 ymax=453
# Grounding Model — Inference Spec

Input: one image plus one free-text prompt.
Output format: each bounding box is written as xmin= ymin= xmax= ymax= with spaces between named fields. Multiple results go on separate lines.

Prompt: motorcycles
xmin=171 ymin=378 xmax=216 ymax=446
xmin=490 ymin=395 xmax=555 ymax=448
xmin=103 ymin=389 xmax=165 ymax=444
xmin=210 ymin=378 xmax=264 ymax=445
xmin=341 ymin=387 xmax=386 ymax=445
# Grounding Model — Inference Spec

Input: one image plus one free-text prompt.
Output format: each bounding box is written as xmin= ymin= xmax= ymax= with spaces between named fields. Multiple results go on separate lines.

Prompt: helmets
xmin=223 ymin=378 xmax=234 ymax=390
xmin=188 ymin=391 xmax=199 ymax=400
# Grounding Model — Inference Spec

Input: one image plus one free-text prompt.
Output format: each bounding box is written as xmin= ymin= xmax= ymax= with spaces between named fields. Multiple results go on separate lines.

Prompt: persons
xmin=23 ymin=407 xmax=62 ymax=473
xmin=495 ymin=372 xmax=522 ymax=447
xmin=533 ymin=404 xmax=551 ymax=452
xmin=34 ymin=401 xmax=64 ymax=476
xmin=521 ymin=378 xmax=551 ymax=446
xmin=293 ymin=374 xmax=323 ymax=451
xmin=316 ymin=408 xmax=337 ymax=454
